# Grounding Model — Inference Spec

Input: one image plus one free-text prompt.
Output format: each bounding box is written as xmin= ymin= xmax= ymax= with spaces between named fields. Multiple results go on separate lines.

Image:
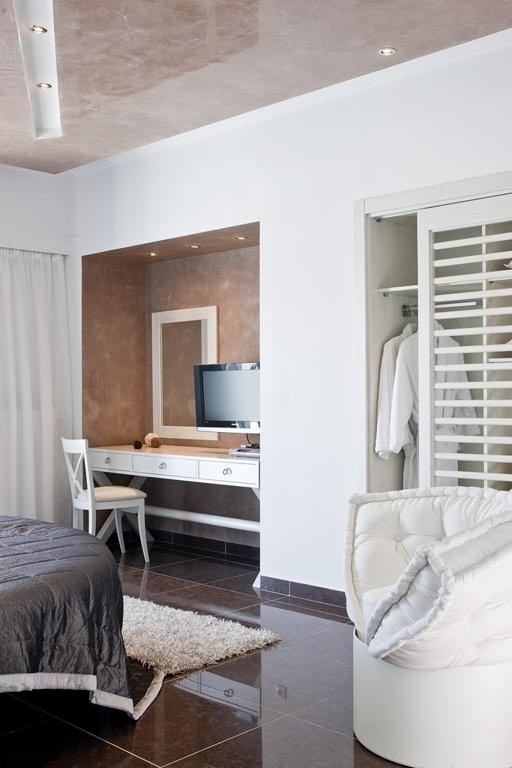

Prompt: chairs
xmin=344 ymin=485 xmax=512 ymax=768
xmin=59 ymin=435 xmax=151 ymax=563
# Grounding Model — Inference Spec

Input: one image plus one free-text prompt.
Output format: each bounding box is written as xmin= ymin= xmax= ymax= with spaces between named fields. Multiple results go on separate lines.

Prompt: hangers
xmin=408 ymin=304 xmax=418 ymax=333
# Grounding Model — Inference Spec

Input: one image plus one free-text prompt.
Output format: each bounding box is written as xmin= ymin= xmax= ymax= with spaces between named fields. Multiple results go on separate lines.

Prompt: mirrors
xmin=151 ymin=304 xmax=220 ymax=441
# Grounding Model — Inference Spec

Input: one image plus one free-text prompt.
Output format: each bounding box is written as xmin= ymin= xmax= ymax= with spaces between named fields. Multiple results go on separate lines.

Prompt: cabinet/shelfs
xmin=351 ymin=170 xmax=512 ymax=490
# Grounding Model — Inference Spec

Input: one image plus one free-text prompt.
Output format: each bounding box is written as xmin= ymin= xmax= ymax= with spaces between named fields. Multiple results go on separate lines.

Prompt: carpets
xmin=120 ymin=595 xmax=282 ymax=676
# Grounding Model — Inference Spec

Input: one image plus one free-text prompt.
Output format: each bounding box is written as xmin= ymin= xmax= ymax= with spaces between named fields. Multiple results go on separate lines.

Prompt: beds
xmin=0 ymin=514 xmax=165 ymax=721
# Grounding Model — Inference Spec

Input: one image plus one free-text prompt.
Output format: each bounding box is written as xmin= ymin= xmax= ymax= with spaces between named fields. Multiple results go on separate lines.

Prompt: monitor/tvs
xmin=192 ymin=361 xmax=261 ymax=456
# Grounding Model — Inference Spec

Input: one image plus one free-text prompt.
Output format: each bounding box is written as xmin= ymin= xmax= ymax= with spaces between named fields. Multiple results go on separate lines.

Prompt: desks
xmin=87 ymin=443 xmax=261 ymax=595
xmin=123 ymin=587 xmax=260 ymax=719
